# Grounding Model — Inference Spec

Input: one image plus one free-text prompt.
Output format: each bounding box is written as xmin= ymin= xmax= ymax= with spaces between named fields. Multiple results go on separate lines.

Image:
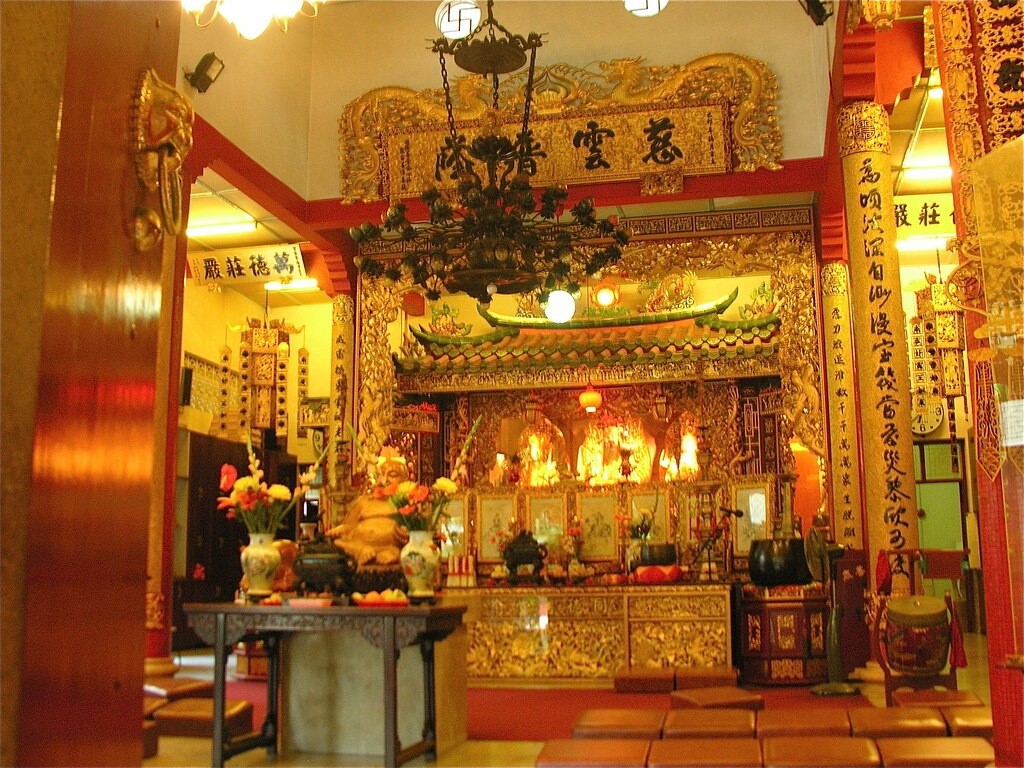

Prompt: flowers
xmin=382 ymin=415 xmax=486 ymax=532
xmin=214 ymin=428 xmax=335 ymax=534
xmin=642 ymin=505 xmax=655 ymax=536
xmin=630 ymin=510 xmax=643 ymax=537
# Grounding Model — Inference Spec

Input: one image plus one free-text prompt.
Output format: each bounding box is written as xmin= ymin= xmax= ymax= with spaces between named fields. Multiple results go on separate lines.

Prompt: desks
xmin=183 ymin=604 xmax=469 ymax=768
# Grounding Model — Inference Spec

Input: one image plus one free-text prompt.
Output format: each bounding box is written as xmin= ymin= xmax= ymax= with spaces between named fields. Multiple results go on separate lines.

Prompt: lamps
xmin=579 ymin=258 xmax=602 ymax=414
xmin=435 ymin=0 xmax=482 ymax=40
xmin=624 ymin=0 xmax=669 ymax=17
xmin=350 ymin=0 xmax=633 ymax=308
xmin=544 ymin=290 xmax=576 ymax=324
xmin=594 ymin=283 xmax=621 ymax=308
xmin=181 ymin=0 xmax=318 ymax=43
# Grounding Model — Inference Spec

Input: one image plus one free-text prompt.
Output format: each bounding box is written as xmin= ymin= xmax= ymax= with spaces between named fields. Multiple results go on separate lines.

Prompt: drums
xmin=884 ymin=595 xmax=952 ymax=678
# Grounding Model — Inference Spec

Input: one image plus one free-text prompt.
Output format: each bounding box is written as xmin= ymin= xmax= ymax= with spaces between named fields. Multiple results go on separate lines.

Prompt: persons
xmin=326 ymin=460 xmax=411 ymax=565
xmin=568 ymin=516 xmax=585 ymax=560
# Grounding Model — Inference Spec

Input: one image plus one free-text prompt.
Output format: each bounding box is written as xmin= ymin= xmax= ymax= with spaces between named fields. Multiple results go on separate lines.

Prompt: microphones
xmin=720 ymin=507 xmax=743 ymax=517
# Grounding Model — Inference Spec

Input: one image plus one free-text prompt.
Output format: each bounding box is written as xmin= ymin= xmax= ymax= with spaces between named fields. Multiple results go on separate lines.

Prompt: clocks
xmin=910 ymin=390 xmax=944 ymax=435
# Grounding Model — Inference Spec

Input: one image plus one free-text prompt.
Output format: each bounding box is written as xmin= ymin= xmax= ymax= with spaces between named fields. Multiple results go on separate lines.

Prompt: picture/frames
xmin=627 ymin=491 xmax=670 ymax=543
xmin=680 ymin=489 xmax=725 ymax=562
xmin=476 ymin=496 xmax=518 ymax=562
xmin=575 ymin=493 xmax=620 ymax=562
xmin=731 ymin=484 xmax=772 ymax=556
xmin=429 ymin=496 xmax=468 ymax=556
xmin=526 ymin=494 xmax=568 ymax=538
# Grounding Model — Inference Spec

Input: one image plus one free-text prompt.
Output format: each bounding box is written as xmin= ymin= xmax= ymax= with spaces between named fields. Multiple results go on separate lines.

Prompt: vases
xmin=628 ymin=538 xmax=641 ymax=572
xmin=243 ymin=535 xmax=281 ymax=595
xmin=640 ymin=538 xmax=650 ymax=566
xmin=401 ymin=532 xmax=442 ymax=596
